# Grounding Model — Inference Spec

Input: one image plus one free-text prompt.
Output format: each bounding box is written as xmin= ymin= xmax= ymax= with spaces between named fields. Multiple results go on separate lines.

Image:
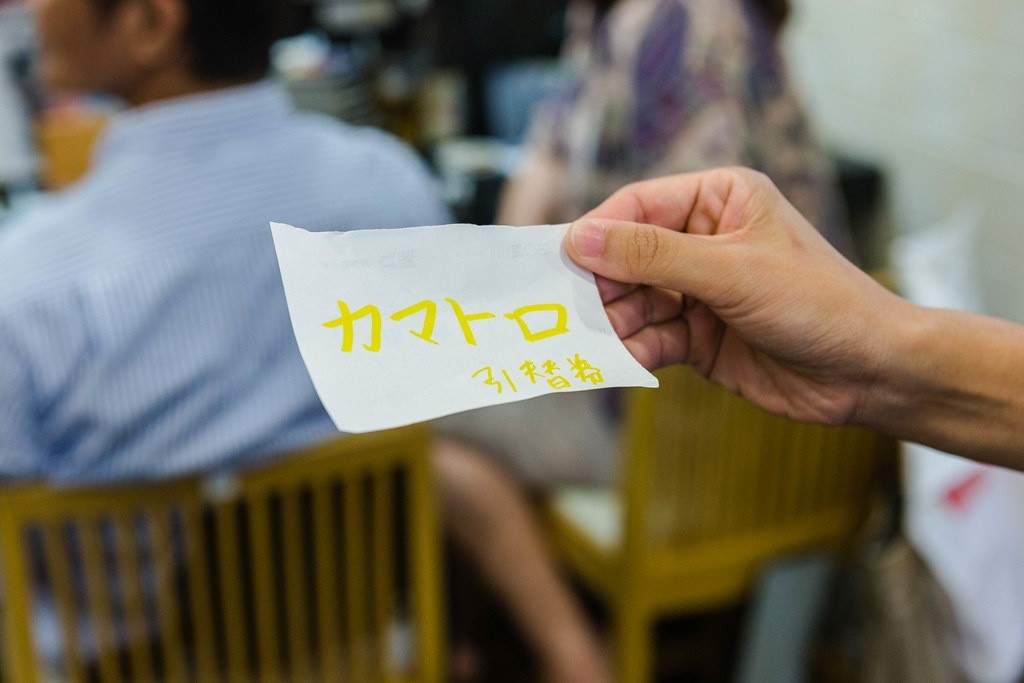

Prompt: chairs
xmin=540 ymin=363 xmax=898 ymax=683
xmin=0 ymin=416 xmax=457 ymax=683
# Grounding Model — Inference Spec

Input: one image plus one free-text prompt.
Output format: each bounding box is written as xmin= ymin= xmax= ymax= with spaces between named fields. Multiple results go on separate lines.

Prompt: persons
xmin=0 ymin=0 xmax=448 ymax=682
xmin=561 ymin=164 xmax=1022 ymax=492
xmin=408 ymin=1 xmax=840 ymax=670
